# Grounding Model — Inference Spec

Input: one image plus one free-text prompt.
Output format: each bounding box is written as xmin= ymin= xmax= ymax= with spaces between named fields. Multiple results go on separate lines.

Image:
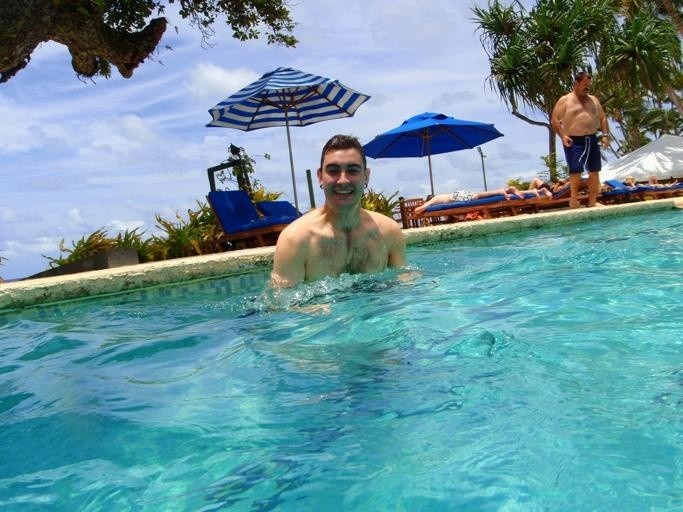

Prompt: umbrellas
xmin=359 ymin=108 xmax=504 ymax=193
xmin=200 ymin=65 xmax=373 ymax=216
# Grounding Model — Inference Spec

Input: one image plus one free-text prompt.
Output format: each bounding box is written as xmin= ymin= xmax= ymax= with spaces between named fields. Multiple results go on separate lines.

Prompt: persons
xmin=547 ymin=70 xmax=616 ymax=209
xmin=412 ymin=173 xmax=682 ymax=221
xmin=265 ymin=133 xmax=422 ymax=311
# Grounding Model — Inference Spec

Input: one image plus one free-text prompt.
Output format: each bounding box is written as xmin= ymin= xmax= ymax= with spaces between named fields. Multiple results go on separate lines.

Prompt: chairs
xmin=205 ymin=189 xmax=306 ymax=250
xmin=397 ymin=176 xmax=682 ymax=230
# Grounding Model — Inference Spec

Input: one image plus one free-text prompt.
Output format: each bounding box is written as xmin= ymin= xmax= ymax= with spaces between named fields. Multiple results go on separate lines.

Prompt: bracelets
xmin=601 ymin=132 xmax=609 ymax=138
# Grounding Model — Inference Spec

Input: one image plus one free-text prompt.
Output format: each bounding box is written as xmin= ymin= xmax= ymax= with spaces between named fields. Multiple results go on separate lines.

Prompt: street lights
xmin=476 ymin=146 xmax=487 ymax=190
